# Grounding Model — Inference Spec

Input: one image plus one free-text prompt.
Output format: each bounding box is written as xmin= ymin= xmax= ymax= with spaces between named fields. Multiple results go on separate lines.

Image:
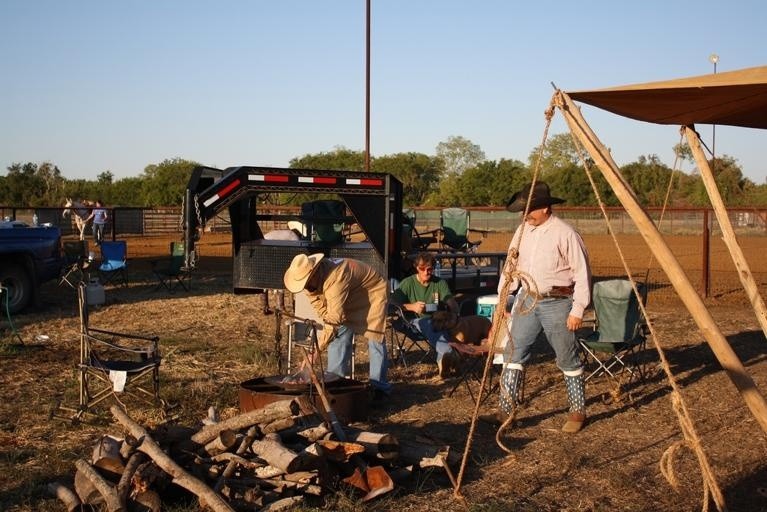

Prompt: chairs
xmin=578 ymin=279 xmax=647 ymax=390
xmin=99 ymin=240 xmax=128 ymax=292
xmin=285 ymin=291 xmax=356 ymax=381
xmin=57 ymin=239 xmax=88 ymax=295
xmin=152 ymin=241 xmax=194 ymax=295
xmin=45 ymin=280 xmax=179 ymax=434
xmin=299 ymin=199 xmax=482 ymax=266
xmin=388 ymin=277 xmax=463 ymax=374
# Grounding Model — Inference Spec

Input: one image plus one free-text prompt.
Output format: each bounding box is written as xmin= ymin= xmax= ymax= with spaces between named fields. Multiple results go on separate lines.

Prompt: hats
xmin=283 ymin=250 xmax=326 ymax=295
xmin=504 ymin=178 xmax=568 ymax=215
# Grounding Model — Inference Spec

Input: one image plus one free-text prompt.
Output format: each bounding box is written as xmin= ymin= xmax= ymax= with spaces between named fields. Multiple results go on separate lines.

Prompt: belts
xmin=547 ymin=286 xmax=574 ymax=298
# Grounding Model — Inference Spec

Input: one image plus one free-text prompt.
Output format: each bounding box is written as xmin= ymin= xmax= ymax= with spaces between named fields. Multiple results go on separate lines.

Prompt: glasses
xmin=417 ymin=266 xmax=435 ymax=273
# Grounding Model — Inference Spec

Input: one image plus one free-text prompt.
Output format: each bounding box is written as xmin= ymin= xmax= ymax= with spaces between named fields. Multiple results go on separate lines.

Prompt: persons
xmin=476 ymin=181 xmax=593 ymax=437
xmin=283 ymin=252 xmax=395 ymax=394
xmin=83 ymin=199 xmax=107 ymax=246
xmin=387 ymin=253 xmax=460 ymax=376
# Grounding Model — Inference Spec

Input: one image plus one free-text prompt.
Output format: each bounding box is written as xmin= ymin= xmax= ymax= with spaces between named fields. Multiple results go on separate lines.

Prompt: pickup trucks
xmin=0 ymin=221 xmax=69 ymax=314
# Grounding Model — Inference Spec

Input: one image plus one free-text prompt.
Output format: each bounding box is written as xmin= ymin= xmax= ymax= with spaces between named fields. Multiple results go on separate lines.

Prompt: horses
xmin=62 ymin=197 xmax=101 ymax=241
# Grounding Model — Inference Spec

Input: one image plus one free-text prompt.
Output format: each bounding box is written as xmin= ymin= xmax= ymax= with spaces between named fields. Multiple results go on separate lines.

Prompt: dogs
xmin=429 ymin=310 xmax=491 ymax=346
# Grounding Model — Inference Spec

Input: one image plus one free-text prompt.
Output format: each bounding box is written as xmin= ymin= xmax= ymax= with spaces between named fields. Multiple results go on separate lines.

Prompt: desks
xmin=449 ymin=342 xmax=526 ymax=407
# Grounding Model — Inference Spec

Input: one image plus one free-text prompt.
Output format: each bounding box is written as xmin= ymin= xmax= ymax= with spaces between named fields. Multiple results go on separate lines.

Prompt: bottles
xmin=435 ymin=261 xmax=440 ymax=277
xmin=32 ymin=214 xmax=38 ymax=225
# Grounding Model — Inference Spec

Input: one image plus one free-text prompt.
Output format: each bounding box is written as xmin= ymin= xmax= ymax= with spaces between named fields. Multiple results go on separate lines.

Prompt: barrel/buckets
xmin=477 ymin=294 xmax=499 ymax=320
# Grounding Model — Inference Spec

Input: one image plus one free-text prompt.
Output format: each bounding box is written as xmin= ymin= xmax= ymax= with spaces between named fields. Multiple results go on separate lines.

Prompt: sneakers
xmin=478 ymin=409 xmax=516 ymax=431
xmin=562 ymin=412 xmax=591 ymax=435
xmin=435 ymin=348 xmax=453 ymax=378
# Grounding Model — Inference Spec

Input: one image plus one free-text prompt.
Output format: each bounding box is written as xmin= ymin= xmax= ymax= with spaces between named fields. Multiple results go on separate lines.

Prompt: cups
xmin=426 ymin=304 xmax=437 ymax=312
